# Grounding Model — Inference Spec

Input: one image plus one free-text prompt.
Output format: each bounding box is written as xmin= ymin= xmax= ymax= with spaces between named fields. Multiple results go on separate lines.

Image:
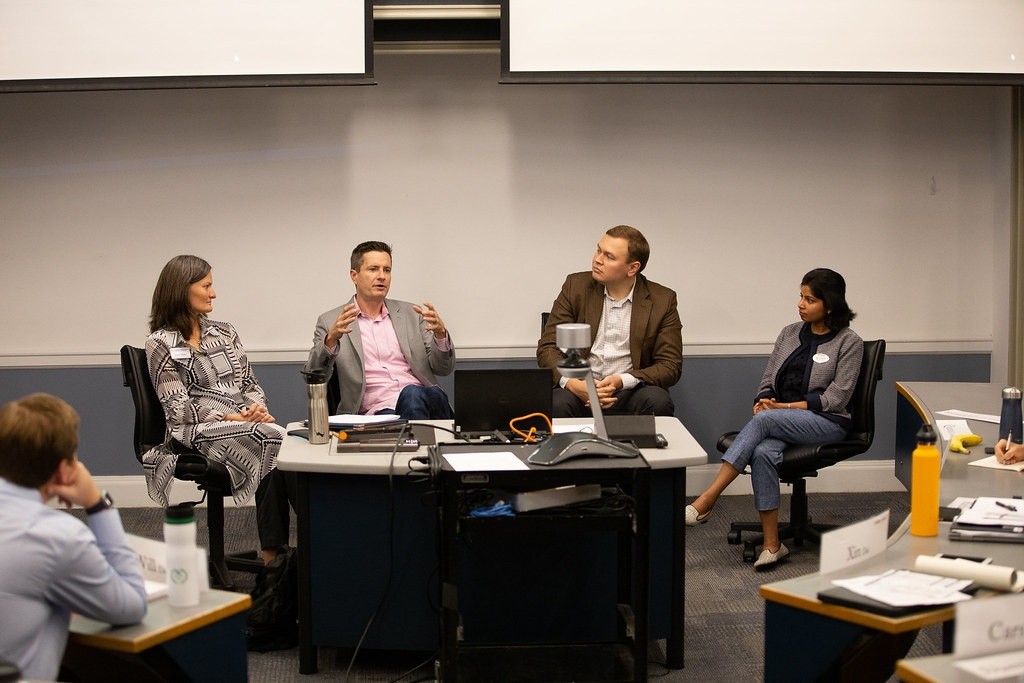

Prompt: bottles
xmin=163 ymin=506 xmax=199 ymax=607
xmin=911 ymin=425 xmax=941 ymax=537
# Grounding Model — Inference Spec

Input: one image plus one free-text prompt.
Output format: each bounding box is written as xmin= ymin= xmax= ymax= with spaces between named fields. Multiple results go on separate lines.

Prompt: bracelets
xmin=788 ymin=403 xmax=790 ymax=408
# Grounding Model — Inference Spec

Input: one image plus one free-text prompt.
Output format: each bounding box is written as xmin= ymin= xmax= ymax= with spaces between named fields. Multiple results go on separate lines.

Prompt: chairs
xmin=720 ymin=338 xmax=888 ymax=553
xmin=120 ymin=345 xmax=297 ymax=621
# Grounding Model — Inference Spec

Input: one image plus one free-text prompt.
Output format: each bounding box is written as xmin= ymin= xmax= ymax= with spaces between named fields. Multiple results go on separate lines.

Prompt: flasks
xmin=999 ymin=387 xmax=1022 ymax=445
xmin=300 ymin=368 xmax=329 ymax=444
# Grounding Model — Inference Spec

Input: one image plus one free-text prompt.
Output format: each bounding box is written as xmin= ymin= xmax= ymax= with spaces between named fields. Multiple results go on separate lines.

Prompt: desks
xmin=67 ymin=580 xmax=253 ymax=683
xmin=276 ymin=405 xmax=712 ymax=683
xmin=758 ymin=380 xmax=1024 ymax=683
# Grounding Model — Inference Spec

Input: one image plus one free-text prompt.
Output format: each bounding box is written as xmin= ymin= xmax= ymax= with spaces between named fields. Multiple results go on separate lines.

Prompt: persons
xmin=303 ymin=240 xmax=456 ymax=420
xmin=0 ymin=392 xmax=148 ymax=683
xmin=536 ymin=226 xmax=683 ymax=417
xmin=685 ymin=268 xmax=864 ymax=567
xmin=995 ymin=439 xmax=1024 ymax=465
xmin=145 ymin=255 xmax=296 ymax=564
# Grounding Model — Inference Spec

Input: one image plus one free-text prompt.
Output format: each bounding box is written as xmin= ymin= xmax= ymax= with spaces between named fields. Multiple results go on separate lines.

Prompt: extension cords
xmin=513 ymin=485 xmax=603 ymax=513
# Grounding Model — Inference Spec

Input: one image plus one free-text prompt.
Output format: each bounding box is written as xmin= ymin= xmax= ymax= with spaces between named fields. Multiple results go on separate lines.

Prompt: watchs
xmin=87 ymin=489 xmax=113 ymax=515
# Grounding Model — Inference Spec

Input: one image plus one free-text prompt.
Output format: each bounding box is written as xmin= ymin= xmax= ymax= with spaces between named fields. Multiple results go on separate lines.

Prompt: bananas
xmin=949 ymin=434 xmax=983 ymax=454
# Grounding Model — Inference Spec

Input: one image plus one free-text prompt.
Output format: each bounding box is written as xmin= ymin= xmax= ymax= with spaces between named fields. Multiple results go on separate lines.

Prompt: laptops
xmin=451 ymin=368 xmax=552 ymax=438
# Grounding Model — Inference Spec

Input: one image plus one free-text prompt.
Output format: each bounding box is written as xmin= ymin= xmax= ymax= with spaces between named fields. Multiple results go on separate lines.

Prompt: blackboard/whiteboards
xmin=0 ymin=42 xmax=1011 ymax=369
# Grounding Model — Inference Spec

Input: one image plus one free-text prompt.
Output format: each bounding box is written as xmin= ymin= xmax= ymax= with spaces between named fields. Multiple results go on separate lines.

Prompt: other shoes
xmin=684 ymin=504 xmax=713 ymax=527
xmin=265 ymin=545 xmax=298 ymax=569
xmin=753 ymin=543 xmax=790 ymax=570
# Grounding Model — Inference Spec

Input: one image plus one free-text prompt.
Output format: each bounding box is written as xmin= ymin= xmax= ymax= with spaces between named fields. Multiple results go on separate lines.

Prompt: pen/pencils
xmin=1003 ymin=429 xmax=1012 ymax=465
xmin=995 ymin=501 xmax=1018 ymax=512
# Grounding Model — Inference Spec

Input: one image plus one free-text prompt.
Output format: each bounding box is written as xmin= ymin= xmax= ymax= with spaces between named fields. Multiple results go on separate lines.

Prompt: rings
xmin=435 ymin=320 xmax=438 ymax=323
